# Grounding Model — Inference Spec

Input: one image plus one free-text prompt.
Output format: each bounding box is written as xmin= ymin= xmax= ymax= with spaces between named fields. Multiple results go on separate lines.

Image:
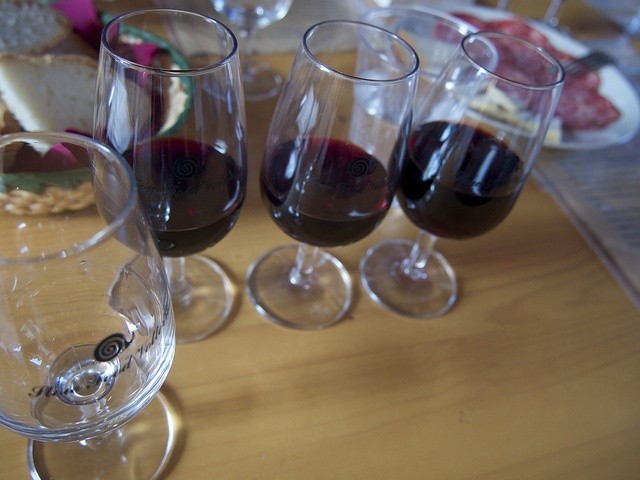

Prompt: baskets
xmin=0 ymin=12 xmax=194 ymax=216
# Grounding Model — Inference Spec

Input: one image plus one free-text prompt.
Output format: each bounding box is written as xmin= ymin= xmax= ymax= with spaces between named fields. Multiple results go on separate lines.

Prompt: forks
xmin=560 ymin=48 xmax=619 ymax=78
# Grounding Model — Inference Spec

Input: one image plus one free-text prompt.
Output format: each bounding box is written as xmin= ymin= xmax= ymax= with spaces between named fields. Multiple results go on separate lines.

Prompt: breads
xmin=0 ymin=54 xmax=152 ymax=155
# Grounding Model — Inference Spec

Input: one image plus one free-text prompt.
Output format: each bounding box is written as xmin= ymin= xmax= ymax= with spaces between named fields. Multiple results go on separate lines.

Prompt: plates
xmin=445 ymin=3 xmax=640 ymax=151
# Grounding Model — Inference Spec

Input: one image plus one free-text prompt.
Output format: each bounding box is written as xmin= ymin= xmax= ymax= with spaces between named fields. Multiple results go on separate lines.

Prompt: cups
xmin=346 ymin=7 xmax=499 ymax=210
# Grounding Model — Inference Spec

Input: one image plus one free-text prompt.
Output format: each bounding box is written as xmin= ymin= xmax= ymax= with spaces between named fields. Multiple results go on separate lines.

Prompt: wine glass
xmin=360 ymin=31 xmax=565 ymax=318
xmin=247 ymin=20 xmax=419 ymax=330
xmin=211 ymin=0 xmax=293 ymax=102
xmin=0 ymin=131 xmax=179 ymax=480
xmin=91 ymin=7 xmax=247 ymax=344
